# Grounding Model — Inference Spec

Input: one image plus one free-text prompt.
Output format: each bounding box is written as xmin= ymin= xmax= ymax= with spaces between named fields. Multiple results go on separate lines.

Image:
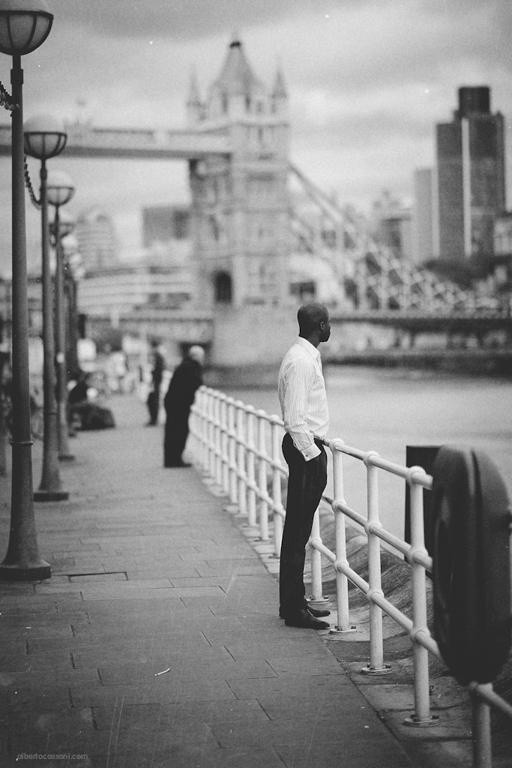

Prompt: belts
xmin=317 ymin=439 xmax=326 ymax=447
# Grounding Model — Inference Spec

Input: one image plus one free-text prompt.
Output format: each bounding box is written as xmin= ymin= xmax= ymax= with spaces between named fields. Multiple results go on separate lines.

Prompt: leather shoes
xmin=284 ymin=605 xmax=330 ymax=630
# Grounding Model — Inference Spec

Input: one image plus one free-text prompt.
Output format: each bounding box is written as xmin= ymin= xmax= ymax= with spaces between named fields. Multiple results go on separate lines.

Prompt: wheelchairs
xmin=3 ymin=379 xmax=44 ymax=442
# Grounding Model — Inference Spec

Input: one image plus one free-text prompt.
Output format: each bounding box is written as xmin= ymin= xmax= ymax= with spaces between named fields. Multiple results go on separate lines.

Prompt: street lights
xmin=20 ymin=114 xmax=72 ymax=499
xmin=0 ymin=1 xmax=56 ymax=581
xmin=46 ymin=169 xmax=89 ymax=461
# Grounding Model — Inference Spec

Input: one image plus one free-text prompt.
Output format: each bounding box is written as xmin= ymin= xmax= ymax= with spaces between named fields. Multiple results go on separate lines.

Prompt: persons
xmin=69 ymin=373 xmax=93 ymax=405
xmin=278 ymin=303 xmax=331 ymax=629
xmin=145 ymin=340 xmax=165 ymax=426
xmin=164 ymin=345 xmax=205 ymax=467
